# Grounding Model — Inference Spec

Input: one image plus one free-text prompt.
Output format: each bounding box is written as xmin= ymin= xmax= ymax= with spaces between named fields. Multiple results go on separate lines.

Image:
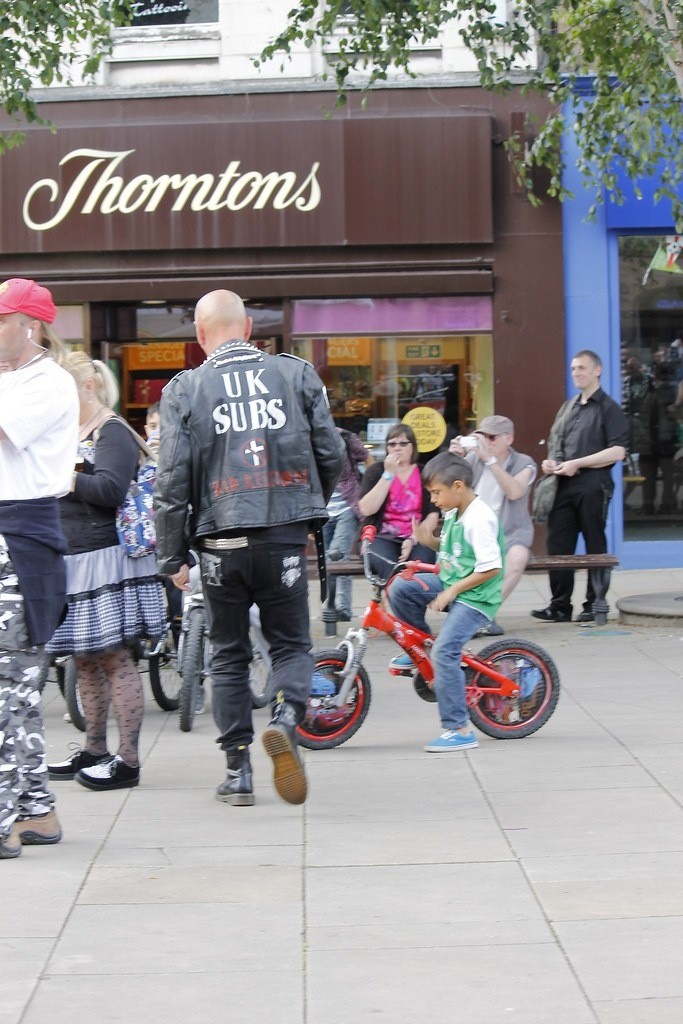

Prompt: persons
xmin=531 ymin=351 xmax=626 ymax=623
xmin=322 ymin=415 xmax=538 ymax=635
xmin=0 ymin=277 xmax=78 ymax=858
xmin=45 ymin=351 xmax=201 ymax=792
xmin=153 ymin=290 xmax=344 ymax=805
xmin=386 ymin=452 xmax=506 ymax=752
xmin=620 ymin=338 xmax=683 ymax=515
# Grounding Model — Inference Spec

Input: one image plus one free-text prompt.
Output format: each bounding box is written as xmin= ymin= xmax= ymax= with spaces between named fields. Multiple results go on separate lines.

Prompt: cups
xmin=146 ymin=429 xmax=160 ymax=446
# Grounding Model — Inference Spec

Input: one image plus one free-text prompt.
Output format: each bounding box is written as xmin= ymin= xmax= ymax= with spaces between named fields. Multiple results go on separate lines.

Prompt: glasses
xmin=486 ymin=434 xmax=508 ymax=441
xmin=386 ymin=441 xmax=411 ymax=448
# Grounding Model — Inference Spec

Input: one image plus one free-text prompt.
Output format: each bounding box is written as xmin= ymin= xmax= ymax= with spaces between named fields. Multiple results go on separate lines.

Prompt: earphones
xmin=27 ymin=329 xmax=32 ymax=339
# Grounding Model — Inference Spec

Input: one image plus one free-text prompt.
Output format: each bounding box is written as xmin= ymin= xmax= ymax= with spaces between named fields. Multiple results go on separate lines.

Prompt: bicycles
xmin=288 ymin=524 xmax=561 ymax=750
xmin=65 ymin=552 xmax=188 ymax=734
xmin=176 ymin=596 xmax=277 ymax=732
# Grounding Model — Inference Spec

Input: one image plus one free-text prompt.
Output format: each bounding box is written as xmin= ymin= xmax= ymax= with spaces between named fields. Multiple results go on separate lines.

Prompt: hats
xmin=473 ymin=415 xmax=515 ymax=436
xmin=0 ymin=278 xmax=56 ymax=323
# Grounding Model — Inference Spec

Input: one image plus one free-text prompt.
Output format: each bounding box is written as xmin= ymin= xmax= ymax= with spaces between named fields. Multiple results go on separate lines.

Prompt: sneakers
xmin=389 ymin=652 xmax=419 ymax=670
xmin=47 ymin=741 xmax=113 ymax=781
xmin=424 ymin=728 xmax=479 ymax=752
xmin=74 ymin=754 xmax=142 ymax=791
xmin=0 ymin=808 xmax=63 ymax=858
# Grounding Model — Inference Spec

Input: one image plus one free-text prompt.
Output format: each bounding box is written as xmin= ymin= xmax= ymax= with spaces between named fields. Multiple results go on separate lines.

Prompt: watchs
xmin=381 ymin=472 xmax=394 ymax=481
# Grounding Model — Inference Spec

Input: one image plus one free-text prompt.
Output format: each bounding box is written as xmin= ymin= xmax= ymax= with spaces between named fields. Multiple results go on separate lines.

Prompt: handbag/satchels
xmin=91 ymin=414 xmax=162 ymax=559
xmin=530 ymin=475 xmax=557 ymax=525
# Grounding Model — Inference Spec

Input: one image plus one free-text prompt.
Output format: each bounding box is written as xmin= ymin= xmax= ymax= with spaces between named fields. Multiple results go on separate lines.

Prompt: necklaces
xmin=79 ymin=407 xmax=107 ymax=434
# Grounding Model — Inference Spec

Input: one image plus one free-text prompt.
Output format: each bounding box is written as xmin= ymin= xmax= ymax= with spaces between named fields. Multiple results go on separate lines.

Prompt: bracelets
xmin=484 ymin=455 xmax=499 ymax=467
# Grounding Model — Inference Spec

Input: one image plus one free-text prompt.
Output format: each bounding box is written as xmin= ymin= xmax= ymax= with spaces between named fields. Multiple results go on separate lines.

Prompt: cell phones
xmin=459 ymin=436 xmax=479 ymax=447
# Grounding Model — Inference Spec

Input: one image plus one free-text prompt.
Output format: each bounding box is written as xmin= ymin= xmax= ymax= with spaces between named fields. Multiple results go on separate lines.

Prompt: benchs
xmin=315 ymin=553 xmax=620 ymax=637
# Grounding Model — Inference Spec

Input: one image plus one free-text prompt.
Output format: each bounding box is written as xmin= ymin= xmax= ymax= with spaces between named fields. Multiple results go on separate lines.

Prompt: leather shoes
xmin=215 ymin=747 xmax=255 ymax=804
xmin=530 ymin=603 xmax=574 ymax=622
xmin=262 ymin=702 xmax=308 ymax=806
xmin=576 ymin=601 xmax=594 ymax=622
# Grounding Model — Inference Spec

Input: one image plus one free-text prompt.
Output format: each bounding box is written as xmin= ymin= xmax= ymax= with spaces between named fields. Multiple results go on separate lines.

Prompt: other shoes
xmin=63 ymin=713 xmax=72 ymax=721
xmin=340 ymin=609 xmax=352 ymax=621
xmin=475 ymin=619 xmax=504 ymax=636
xmin=193 ymin=686 xmax=208 ymax=715
